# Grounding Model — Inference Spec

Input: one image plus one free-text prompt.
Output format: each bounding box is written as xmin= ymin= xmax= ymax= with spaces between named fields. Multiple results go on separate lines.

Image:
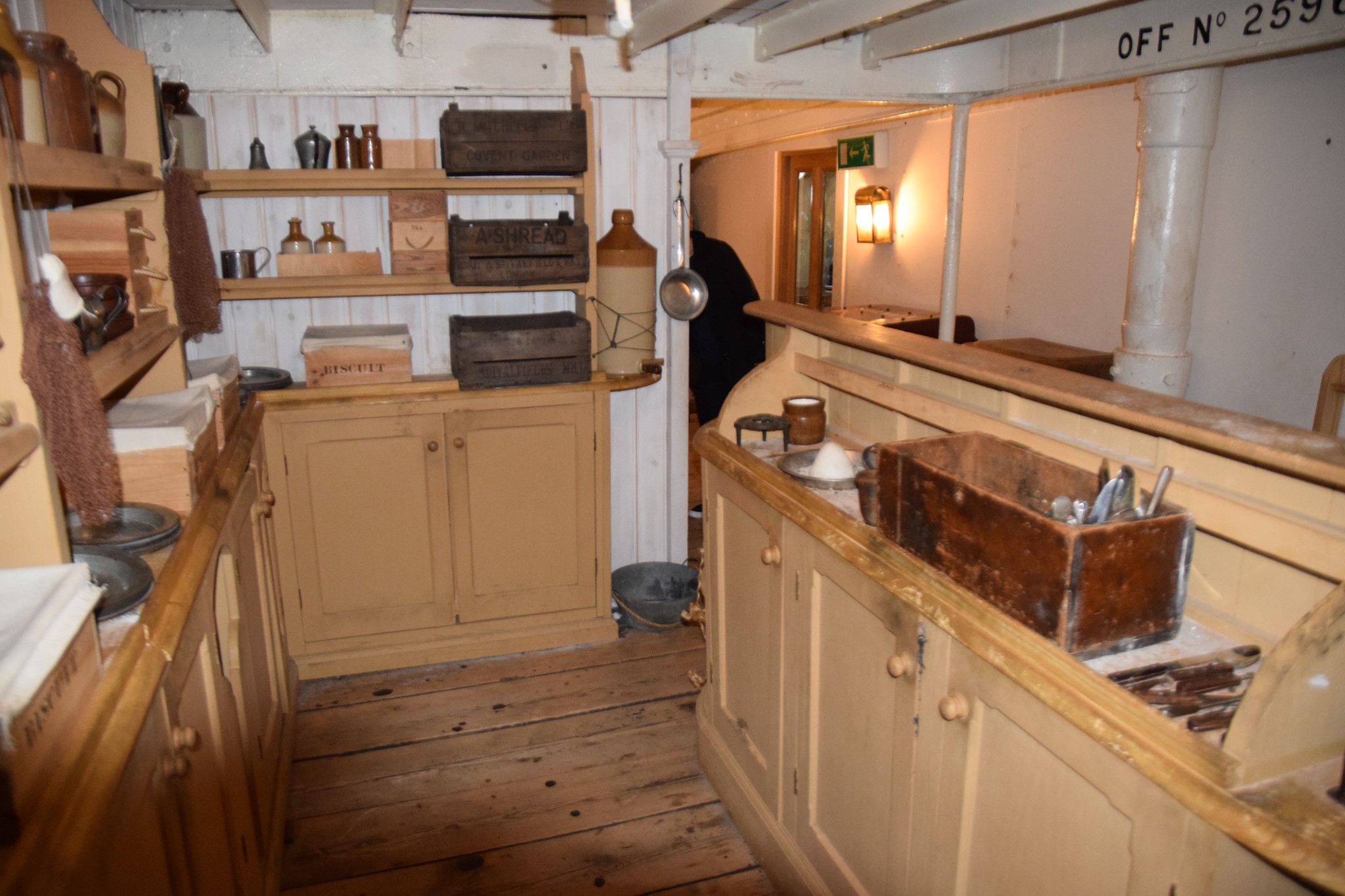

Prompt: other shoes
xmin=689 ymin=503 xmax=703 ymax=518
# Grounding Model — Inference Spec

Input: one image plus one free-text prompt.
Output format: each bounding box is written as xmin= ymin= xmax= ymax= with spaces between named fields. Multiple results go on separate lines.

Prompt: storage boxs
xmin=301 ymin=324 xmax=413 ymax=386
xmin=859 ymin=434 xmax=1199 ymax=662
xmin=447 ymin=211 xmax=589 ymax=286
xmin=387 ymin=190 xmax=449 ymax=274
xmin=439 ymin=102 xmax=588 ymax=175
xmin=448 ymin=311 xmax=592 ymax=390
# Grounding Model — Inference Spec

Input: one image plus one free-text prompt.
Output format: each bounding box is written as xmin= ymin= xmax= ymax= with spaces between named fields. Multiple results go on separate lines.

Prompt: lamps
xmin=855 ymin=185 xmax=894 ymax=244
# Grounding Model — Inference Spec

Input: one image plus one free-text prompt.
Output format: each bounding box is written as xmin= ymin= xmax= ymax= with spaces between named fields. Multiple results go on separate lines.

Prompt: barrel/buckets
xmin=611 ymin=558 xmax=700 ymax=631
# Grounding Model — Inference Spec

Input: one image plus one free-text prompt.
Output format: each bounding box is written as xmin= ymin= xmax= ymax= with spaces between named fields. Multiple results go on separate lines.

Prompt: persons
xmin=688 ymin=230 xmax=766 ymax=520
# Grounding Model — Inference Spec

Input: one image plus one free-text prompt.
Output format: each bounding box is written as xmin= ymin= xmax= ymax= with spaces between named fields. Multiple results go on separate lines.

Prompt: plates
xmin=68 ymin=545 xmax=155 ymax=620
xmin=64 ymin=502 xmax=184 ymax=556
xmin=236 ymin=366 xmax=292 ymax=405
xmin=777 ymin=449 xmax=881 ymax=489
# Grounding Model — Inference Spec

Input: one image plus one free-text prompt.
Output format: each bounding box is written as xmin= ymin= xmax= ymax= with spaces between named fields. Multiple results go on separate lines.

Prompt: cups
xmin=855 ymin=468 xmax=879 ymax=526
xmin=1107 ymin=645 xmax=1260 ymax=741
xmin=220 ymin=246 xmax=271 ymax=280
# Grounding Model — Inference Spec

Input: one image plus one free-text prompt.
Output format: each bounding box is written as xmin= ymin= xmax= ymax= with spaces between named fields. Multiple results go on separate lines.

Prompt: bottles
xmin=314 ymin=221 xmax=346 ymax=253
xmin=1 ymin=0 xmax=128 ymax=156
xmin=335 ymin=124 xmax=383 ymax=169
xmin=596 ymin=209 xmax=657 ymax=379
xmin=295 ymin=125 xmax=331 ymax=168
xmin=248 ymin=136 xmax=270 ymax=169
xmin=279 ymin=217 xmax=313 ymax=254
xmin=781 ymin=395 xmax=827 ymax=445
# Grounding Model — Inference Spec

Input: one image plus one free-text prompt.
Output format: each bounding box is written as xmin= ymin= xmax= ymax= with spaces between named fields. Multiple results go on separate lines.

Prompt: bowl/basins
xmin=69 ymin=274 xmax=128 ymax=320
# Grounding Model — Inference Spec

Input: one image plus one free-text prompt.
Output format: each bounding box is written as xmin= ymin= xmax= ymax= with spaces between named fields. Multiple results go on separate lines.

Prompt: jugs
xmin=78 ymin=285 xmax=127 ymax=350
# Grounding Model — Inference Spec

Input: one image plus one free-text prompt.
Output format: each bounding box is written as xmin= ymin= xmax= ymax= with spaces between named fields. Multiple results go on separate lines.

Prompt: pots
xmin=661 ymin=198 xmax=708 ymax=321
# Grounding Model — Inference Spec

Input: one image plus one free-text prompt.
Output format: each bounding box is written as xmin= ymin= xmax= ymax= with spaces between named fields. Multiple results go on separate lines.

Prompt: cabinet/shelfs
xmin=0 ymin=0 xmax=611 ymax=896
xmin=690 ymin=300 xmax=1345 ymax=896
